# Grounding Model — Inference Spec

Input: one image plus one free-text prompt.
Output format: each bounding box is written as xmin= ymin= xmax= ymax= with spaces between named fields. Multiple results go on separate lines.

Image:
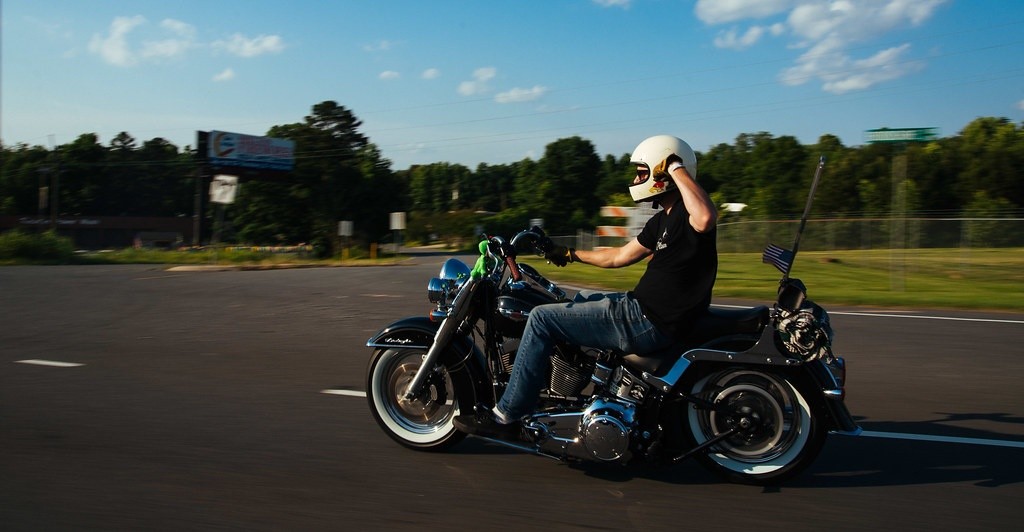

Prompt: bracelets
xmin=668 ymin=163 xmax=686 ymax=174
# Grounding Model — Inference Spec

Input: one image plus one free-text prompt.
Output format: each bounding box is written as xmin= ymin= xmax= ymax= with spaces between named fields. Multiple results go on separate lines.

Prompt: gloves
xmin=653 ymin=154 xmax=683 ymax=182
xmin=545 ymin=246 xmax=575 ymax=267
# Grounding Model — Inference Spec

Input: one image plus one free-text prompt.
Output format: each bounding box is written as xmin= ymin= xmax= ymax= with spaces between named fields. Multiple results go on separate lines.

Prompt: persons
xmin=453 ymin=135 xmax=718 ymax=443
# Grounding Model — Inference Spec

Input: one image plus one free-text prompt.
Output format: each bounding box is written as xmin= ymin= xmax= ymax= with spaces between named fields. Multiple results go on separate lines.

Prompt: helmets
xmin=627 ymin=135 xmax=697 ymax=203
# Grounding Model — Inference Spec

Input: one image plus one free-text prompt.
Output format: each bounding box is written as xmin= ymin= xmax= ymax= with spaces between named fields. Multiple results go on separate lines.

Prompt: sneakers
xmin=452 ymin=407 xmax=518 ymax=442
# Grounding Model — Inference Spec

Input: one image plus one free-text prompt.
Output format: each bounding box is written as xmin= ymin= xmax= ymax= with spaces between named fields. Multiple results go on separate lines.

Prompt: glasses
xmin=636 ymin=170 xmax=649 ymax=182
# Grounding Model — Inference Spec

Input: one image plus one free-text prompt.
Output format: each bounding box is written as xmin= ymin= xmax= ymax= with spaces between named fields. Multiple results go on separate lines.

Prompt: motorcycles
xmin=365 ymin=156 xmax=863 ymax=486
